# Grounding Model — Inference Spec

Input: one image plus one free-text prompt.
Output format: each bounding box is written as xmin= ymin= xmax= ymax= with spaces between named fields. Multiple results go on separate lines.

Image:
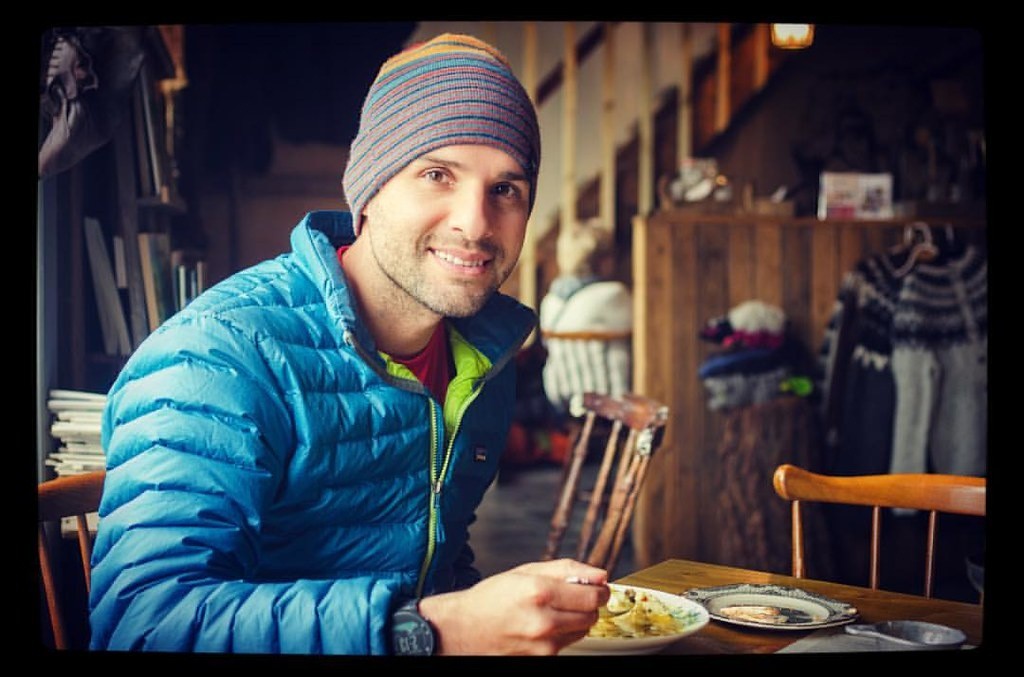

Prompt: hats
xmin=341 ymin=31 xmax=543 ymax=238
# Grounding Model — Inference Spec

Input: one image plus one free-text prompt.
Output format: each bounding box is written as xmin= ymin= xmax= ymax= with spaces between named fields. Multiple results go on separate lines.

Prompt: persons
xmin=87 ymin=33 xmax=612 ymax=654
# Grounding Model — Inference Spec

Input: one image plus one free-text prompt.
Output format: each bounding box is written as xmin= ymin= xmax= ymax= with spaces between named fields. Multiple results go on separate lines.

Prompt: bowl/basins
xmin=845 ymin=621 xmax=967 ymax=649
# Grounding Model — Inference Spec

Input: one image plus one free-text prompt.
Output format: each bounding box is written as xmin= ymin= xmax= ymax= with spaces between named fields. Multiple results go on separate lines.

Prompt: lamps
xmin=771 ymin=23 xmax=815 ymax=50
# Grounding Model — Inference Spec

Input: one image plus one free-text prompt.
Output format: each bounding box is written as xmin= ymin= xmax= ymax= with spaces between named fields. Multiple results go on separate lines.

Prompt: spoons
xmin=568 ymin=576 xmax=637 ymax=615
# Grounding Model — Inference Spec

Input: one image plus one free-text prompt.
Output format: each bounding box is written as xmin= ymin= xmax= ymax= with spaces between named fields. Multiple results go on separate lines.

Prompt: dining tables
xmin=556 ymin=560 xmax=984 ymax=656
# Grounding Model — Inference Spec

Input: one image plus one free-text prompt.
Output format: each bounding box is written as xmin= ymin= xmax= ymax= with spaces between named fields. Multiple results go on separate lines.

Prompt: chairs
xmin=534 ymin=390 xmax=670 ymax=580
xmin=38 ymin=470 xmax=107 ymax=649
xmin=771 ymin=460 xmax=988 ymax=604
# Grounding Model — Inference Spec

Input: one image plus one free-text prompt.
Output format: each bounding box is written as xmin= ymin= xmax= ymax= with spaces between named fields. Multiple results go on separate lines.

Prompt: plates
xmin=557 ymin=582 xmax=712 ymax=654
xmin=680 ymin=584 xmax=859 ymax=630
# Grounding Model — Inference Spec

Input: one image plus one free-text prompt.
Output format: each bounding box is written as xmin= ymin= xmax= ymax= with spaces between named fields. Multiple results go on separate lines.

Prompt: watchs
xmin=388 ymin=596 xmax=436 ymax=654
